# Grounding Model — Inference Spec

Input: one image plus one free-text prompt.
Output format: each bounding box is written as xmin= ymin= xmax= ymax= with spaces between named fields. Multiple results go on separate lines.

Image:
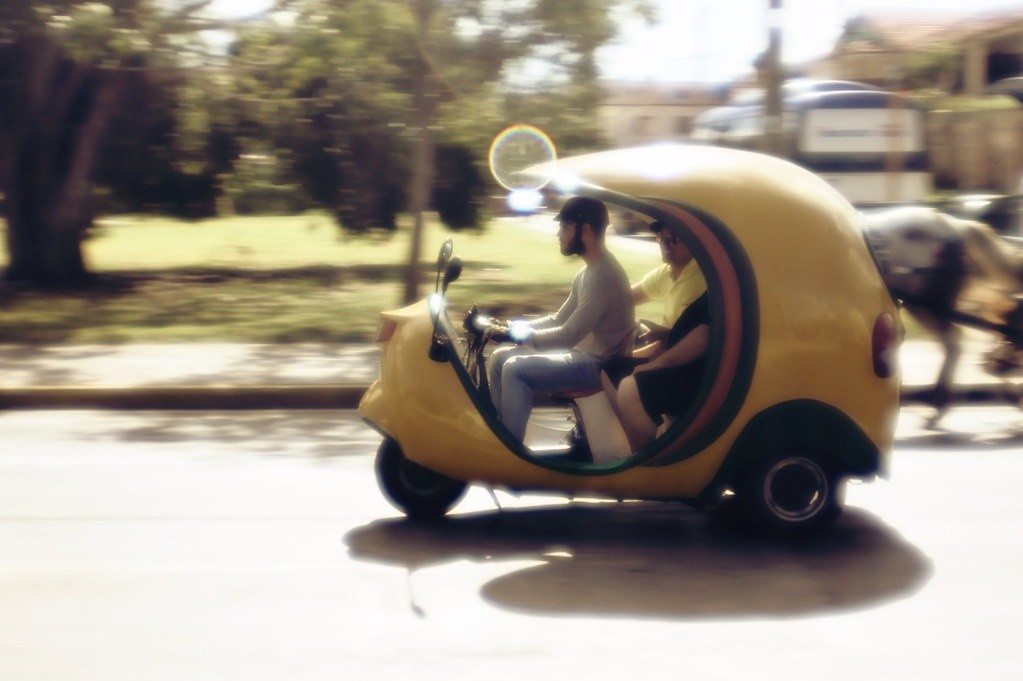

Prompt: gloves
xmin=489 ymin=319 xmax=508 ymax=327
xmin=482 ymin=327 xmax=513 ymax=342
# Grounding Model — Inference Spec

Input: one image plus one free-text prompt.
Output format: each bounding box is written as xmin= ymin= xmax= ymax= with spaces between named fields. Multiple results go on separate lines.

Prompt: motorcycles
xmin=352 ymin=143 xmax=907 ymax=537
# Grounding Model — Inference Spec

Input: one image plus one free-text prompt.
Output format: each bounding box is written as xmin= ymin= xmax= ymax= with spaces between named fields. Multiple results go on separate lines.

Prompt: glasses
xmin=655 ymin=232 xmax=682 ymax=245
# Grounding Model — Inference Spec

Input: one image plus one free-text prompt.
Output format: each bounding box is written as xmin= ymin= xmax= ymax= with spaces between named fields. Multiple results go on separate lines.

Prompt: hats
xmin=649 ymin=220 xmax=665 ymax=232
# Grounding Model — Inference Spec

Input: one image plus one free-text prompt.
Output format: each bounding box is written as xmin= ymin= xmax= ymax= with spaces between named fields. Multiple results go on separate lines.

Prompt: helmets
xmin=552 ymin=197 xmax=609 ymax=224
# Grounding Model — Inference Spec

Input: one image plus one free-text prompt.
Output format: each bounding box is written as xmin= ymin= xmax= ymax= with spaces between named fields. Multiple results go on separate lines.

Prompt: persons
xmin=599 ymin=222 xmax=713 ymax=453
xmin=489 ymin=196 xmax=632 ymax=448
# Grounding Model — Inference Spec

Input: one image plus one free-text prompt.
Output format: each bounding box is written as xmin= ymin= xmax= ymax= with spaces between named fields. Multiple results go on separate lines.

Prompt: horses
xmin=861 ymin=203 xmax=1023 ymax=407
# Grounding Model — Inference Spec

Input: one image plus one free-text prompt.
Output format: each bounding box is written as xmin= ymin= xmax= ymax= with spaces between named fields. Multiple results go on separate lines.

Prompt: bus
xmin=687 ymin=78 xmax=932 ymax=212
